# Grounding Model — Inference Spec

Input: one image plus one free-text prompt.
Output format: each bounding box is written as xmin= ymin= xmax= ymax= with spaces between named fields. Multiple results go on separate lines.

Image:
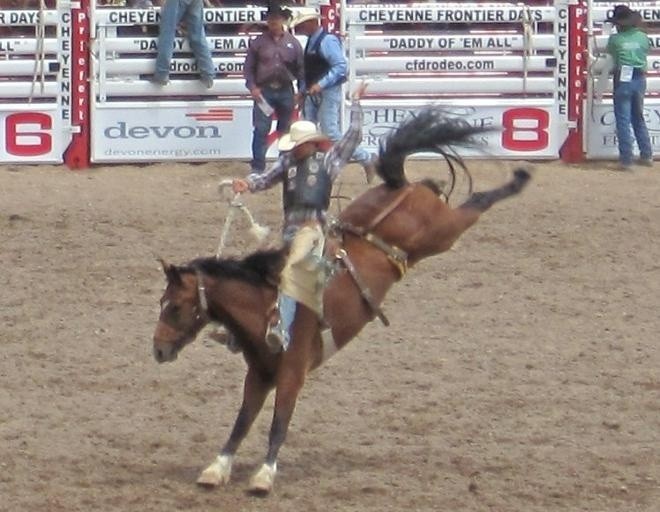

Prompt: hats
xmin=278 ymin=121 xmax=321 ymax=151
xmin=604 ymin=5 xmax=642 ymax=26
xmin=288 ymin=7 xmax=320 ymax=28
xmin=264 ymin=2 xmax=288 ymax=18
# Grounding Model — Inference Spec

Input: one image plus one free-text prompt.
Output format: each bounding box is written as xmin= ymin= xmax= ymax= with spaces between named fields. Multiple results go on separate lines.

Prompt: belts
xmin=263 ymin=81 xmax=291 ymax=90
xmin=633 ymin=72 xmax=645 ymax=75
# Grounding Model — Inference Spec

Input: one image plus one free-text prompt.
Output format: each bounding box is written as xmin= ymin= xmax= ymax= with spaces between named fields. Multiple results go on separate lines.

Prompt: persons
xmin=606 ymin=5 xmax=655 ymax=170
xmin=147 ymin=0 xmax=216 ymax=89
xmin=244 ymin=6 xmax=378 ymax=184
xmin=232 ymin=77 xmax=369 ymax=353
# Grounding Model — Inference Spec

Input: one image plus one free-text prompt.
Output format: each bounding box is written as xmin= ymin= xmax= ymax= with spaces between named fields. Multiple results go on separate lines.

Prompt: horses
xmin=151 ymin=105 xmax=537 ymax=496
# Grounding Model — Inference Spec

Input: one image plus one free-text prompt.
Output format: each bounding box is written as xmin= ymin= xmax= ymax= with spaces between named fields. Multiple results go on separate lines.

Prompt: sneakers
xmin=607 ymin=158 xmax=653 ymax=174
xmin=364 ymin=152 xmax=379 ymax=184
xmin=265 ymin=317 xmax=290 ymax=354
xmin=152 ymin=77 xmax=213 ymax=90
